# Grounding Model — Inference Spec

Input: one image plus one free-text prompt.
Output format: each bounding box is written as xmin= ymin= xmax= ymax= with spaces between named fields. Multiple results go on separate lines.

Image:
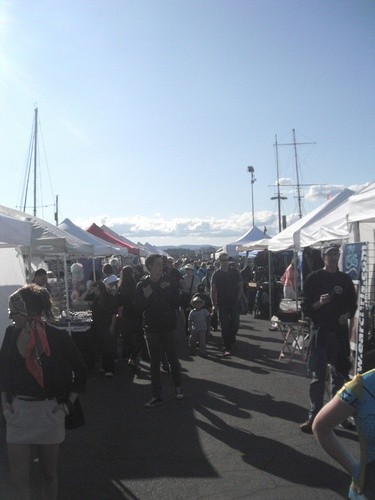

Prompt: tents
xmin=0 ymin=205 xmax=172 ymax=337
xmin=215 ymin=182 xmax=375 ymax=352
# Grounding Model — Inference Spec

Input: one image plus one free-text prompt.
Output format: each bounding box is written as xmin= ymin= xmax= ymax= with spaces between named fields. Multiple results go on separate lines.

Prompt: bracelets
xmin=63 ymin=397 xmax=73 ymax=412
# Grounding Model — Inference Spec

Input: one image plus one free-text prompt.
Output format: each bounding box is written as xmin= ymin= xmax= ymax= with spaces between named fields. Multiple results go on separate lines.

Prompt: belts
xmin=14 ymin=395 xmax=52 ymax=401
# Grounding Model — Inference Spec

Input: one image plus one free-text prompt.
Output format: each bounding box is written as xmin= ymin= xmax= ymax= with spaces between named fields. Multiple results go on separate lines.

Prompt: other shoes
xmin=339 ymin=420 xmax=352 ymax=428
xmin=105 ymin=370 xmax=113 ymax=376
xmin=145 ymin=397 xmax=162 ymax=408
xmin=224 ymin=351 xmax=231 ymax=355
xmin=128 ymin=359 xmax=138 ymax=370
xmin=299 ymin=420 xmax=312 ymax=431
xmin=176 ymin=387 xmax=184 ymax=397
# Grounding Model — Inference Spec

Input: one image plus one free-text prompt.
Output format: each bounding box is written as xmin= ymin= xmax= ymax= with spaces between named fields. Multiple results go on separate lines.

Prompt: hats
xmin=322 ymin=243 xmax=340 ymax=254
xmin=190 ymin=297 xmax=204 ymax=308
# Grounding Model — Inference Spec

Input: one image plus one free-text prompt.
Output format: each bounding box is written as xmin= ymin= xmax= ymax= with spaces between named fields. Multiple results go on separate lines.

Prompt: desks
xmin=274 ymin=321 xmax=306 ymax=362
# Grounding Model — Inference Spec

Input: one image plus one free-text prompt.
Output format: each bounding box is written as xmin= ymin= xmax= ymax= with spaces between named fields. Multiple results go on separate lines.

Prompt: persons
xmin=312 ymin=368 xmax=375 ymax=500
xmin=1 ymin=284 xmax=77 ymax=500
xmin=72 ymin=252 xmax=256 ymax=377
xmin=280 ymin=256 xmax=299 ymax=301
xmin=131 ymin=254 xmax=187 ymax=406
xmin=30 ymin=269 xmax=47 ymax=287
xmin=298 ymin=242 xmax=358 ymax=431
